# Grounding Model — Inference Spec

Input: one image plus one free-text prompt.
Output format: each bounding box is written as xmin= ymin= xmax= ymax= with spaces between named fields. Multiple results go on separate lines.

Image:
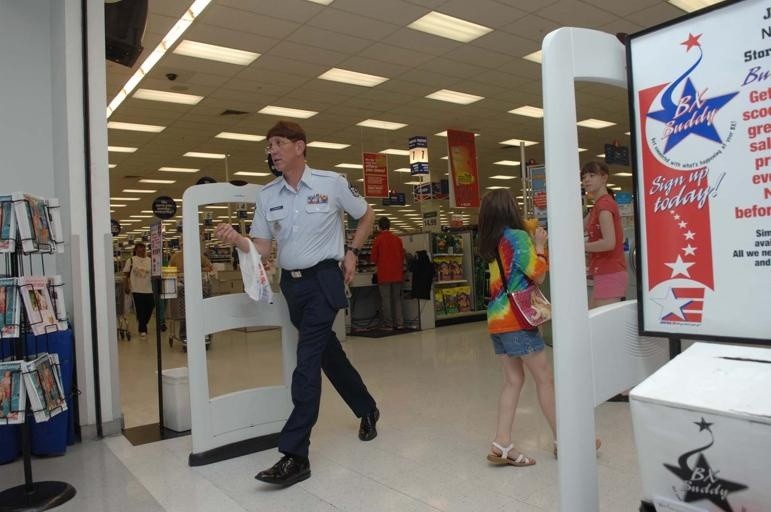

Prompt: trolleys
xmin=115 ymin=275 xmax=131 ymax=342
xmin=165 ymin=274 xmax=215 ymax=354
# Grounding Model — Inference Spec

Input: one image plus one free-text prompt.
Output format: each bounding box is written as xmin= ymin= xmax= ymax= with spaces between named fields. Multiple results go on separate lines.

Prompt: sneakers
xmin=140 ymin=332 xmax=147 ymax=340
xmin=379 ymin=325 xmax=405 ymax=331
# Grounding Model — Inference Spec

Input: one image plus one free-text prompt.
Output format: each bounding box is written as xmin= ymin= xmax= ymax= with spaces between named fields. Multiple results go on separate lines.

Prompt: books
xmin=0 ymin=195 xmax=69 ymax=427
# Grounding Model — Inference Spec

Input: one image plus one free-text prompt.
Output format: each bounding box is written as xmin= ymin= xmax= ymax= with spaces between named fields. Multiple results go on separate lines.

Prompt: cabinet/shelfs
xmin=626 ymin=341 xmax=771 ymax=512
xmin=431 ymin=252 xmax=473 ymax=320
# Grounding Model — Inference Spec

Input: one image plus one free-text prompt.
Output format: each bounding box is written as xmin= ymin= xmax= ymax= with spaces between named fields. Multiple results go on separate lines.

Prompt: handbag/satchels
xmin=508 ymin=284 xmax=553 ymax=331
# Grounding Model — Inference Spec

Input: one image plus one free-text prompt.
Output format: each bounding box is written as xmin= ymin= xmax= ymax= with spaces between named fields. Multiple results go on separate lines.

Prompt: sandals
xmin=486 ymin=442 xmax=536 ymax=467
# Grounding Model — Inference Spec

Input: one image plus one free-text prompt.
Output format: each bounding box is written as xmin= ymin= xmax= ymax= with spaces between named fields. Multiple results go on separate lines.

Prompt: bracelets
xmin=348 ymin=247 xmax=361 ymax=256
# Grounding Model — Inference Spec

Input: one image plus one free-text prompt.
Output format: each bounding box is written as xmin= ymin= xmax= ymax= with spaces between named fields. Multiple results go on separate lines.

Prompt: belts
xmin=255 ymin=457 xmax=310 ymax=485
xmin=282 ymin=261 xmax=320 ymax=280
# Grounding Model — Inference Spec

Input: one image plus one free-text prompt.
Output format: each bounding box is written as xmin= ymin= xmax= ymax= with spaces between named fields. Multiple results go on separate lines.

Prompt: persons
xmin=168 ymin=235 xmax=213 ymax=353
xmin=122 ymin=243 xmax=156 ymax=337
xmin=477 ymin=188 xmax=558 ymax=467
xmin=580 ymin=160 xmax=631 ymax=401
xmin=212 ymin=119 xmax=380 ymax=486
xmin=370 ymin=216 xmax=405 ymax=331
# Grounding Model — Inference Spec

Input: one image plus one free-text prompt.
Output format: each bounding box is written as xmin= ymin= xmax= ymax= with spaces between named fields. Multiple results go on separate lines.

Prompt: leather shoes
xmin=358 ymin=407 xmax=379 ymax=440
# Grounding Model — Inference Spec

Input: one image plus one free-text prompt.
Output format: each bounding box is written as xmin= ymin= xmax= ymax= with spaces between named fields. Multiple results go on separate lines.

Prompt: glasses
xmin=263 ymin=138 xmax=299 ymax=154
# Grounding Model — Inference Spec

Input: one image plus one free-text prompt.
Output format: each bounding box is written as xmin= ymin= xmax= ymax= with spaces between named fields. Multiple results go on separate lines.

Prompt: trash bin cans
xmin=155 ymin=367 xmax=192 ymax=433
